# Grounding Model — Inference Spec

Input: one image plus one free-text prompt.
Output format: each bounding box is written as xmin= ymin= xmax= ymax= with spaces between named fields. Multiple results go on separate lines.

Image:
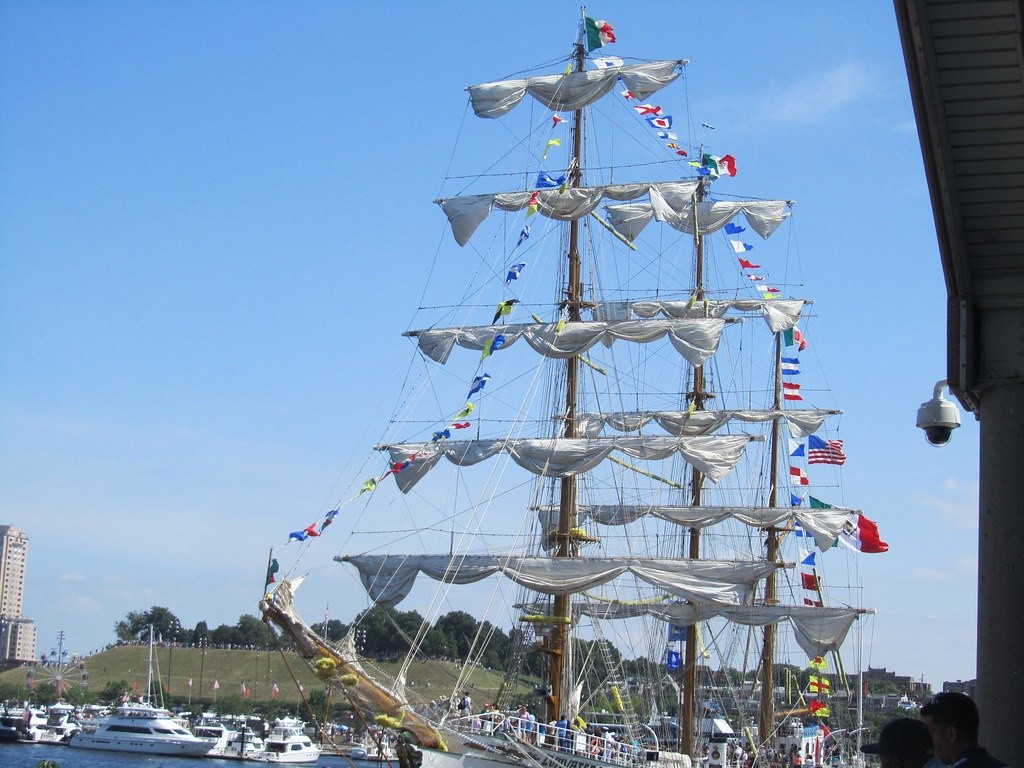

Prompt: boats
xmin=192 ymin=722 xmax=263 ymax=759
xmin=0 ymin=707 xmax=80 ymax=745
xmin=348 ymin=737 xmax=400 ymax=761
xmin=247 ymin=726 xmax=323 ymax=763
xmin=69 ymin=703 xmax=218 ymax=756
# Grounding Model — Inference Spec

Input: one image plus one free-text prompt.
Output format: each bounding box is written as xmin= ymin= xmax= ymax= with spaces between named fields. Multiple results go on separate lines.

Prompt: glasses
xmin=931 ymin=691 xmax=956 ymax=720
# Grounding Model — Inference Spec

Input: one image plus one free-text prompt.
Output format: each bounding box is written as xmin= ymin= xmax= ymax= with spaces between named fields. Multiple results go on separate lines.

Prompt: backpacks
xmin=458 ymin=696 xmax=468 ymax=710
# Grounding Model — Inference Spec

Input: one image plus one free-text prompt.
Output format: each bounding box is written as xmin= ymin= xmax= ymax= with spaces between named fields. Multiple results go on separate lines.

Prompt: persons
xmin=859 ymin=692 xmax=1009 ymax=768
xmin=459 ymin=690 xmax=816 ymax=768
xmin=69 ymin=642 xmax=290 ymax=663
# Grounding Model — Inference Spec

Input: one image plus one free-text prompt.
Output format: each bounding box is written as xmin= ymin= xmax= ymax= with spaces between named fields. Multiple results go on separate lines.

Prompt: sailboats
xmin=258 ymin=5 xmax=888 ymax=767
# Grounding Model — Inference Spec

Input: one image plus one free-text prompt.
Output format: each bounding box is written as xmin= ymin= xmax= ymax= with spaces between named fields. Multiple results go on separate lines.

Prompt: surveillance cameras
xmin=915 ymin=399 xmax=961 ymax=447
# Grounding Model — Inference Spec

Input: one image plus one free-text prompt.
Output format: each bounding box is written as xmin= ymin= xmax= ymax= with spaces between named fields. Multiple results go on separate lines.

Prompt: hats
xmin=860 ymin=719 xmax=934 ymax=758
xmin=485 ymin=703 xmax=489 ymax=707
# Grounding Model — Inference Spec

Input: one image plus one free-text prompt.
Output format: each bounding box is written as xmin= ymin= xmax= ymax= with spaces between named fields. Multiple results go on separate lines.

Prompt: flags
xmin=791 ymin=493 xmax=889 ymax=669
xmin=266 ymin=560 xmax=280 ymax=586
xmin=810 ymin=676 xmax=830 ymax=717
xmin=535 ymin=114 xmax=569 ymax=187
xmin=213 ymin=680 xmax=220 ymax=689
xmin=297 ymin=680 xmax=332 ymax=695
xmin=241 ymin=681 xmax=250 ymax=696
xmin=782 ymin=356 xmax=846 ymax=485
xmin=492 ymin=192 xmax=539 ymax=326
xmin=286 ymin=454 xmax=417 ymax=545
xmin=586 ymin=16 xmax=616 ymax=53
xmin=710 ymin=198 xmax=808 ymax=351
xmin=132 ymin=681 xmax=138 ymax=691
xmin=431 ymin=335 xmax=506 ymax=443
xmin=188 ymin=678 xmax=192 ymax=686
xmin=272 ymin=683 xmax=279 ymax=699
xmin=593 ymin=56 xmax=737 ymax=183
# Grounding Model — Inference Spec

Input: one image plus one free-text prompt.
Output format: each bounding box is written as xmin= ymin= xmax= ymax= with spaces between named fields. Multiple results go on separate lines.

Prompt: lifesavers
xmin=711 ymin=750 xmax=720 ymax=760
xmin=373 ymin=730 xmax=385 ymax=742
xmin=590 ymin=738 xmax=602 ymax=754
